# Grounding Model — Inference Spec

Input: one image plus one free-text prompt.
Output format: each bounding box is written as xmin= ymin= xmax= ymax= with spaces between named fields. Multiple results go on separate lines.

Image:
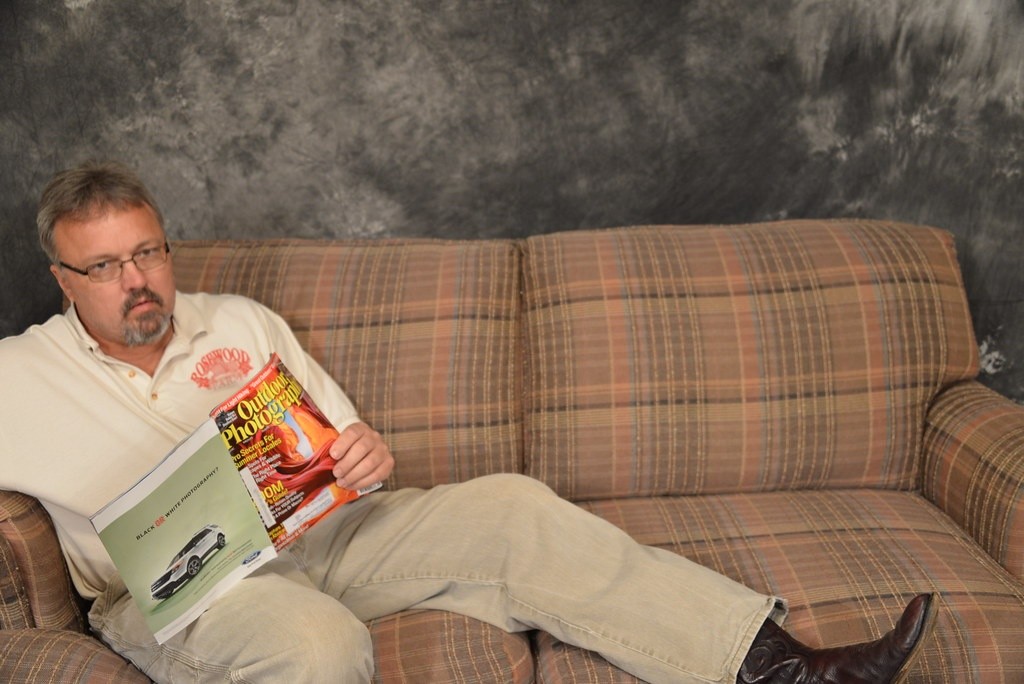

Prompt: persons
xmin=0 ymin=159 xmax=942 ymax=684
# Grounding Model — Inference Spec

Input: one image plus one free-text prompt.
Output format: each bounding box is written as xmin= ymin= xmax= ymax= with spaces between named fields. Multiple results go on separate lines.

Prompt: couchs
xmin=0 ymin=212 xmax=1024 ymax=683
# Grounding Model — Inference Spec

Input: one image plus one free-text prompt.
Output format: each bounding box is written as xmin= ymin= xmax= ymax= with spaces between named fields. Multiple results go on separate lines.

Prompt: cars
xmin=149 ymin=523 xmax=226 ymax=599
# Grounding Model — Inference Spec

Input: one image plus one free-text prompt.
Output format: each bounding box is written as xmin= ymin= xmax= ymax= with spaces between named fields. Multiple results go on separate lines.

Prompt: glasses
xmin=59 ymin=242 xmax=170 ymax=283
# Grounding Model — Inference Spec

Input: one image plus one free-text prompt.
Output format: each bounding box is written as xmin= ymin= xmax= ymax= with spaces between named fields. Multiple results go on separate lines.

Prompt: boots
xmin=739 ymin=592 xmax=941 ymax=684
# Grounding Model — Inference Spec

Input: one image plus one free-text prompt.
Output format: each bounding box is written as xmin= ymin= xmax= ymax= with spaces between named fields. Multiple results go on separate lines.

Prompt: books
xmin=88 ymin=353 xmax=383 ymax=647
